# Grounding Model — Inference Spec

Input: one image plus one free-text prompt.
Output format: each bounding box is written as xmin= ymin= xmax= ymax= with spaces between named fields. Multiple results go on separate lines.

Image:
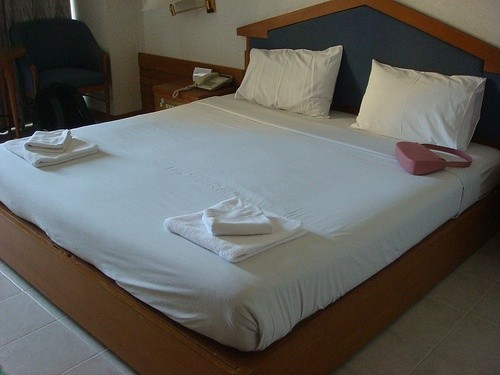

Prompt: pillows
xmin=233 ymin=44 xmax=343 ymax=119
xmin=350 ymin=58 xmax=488 ymax=153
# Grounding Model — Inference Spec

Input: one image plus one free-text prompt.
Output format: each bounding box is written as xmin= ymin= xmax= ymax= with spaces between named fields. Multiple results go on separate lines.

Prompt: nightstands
xmin=150 ymin=78 xmax=238 ymax=112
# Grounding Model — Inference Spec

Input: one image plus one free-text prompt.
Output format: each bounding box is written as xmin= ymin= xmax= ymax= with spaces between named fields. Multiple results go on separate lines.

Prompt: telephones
xmin=195 ymin=72 xmax=233 ymax=91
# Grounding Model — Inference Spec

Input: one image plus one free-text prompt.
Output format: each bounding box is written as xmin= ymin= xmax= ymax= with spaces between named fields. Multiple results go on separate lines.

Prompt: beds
xmin=0 ymin=88 xmax=500 ymax=375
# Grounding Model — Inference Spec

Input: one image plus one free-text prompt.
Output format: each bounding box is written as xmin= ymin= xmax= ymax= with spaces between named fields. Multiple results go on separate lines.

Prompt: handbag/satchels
xmin=395 ymin=140 xmax=473 ymax=176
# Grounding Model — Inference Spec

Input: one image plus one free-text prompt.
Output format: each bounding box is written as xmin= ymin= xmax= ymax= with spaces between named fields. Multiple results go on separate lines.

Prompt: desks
xmin=0 ymin=44 xmax=28 ymax=142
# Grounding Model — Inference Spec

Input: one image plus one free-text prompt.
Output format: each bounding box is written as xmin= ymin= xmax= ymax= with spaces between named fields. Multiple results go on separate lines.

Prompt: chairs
xmin=8 ymin=18 xmax=113 ymax=134
